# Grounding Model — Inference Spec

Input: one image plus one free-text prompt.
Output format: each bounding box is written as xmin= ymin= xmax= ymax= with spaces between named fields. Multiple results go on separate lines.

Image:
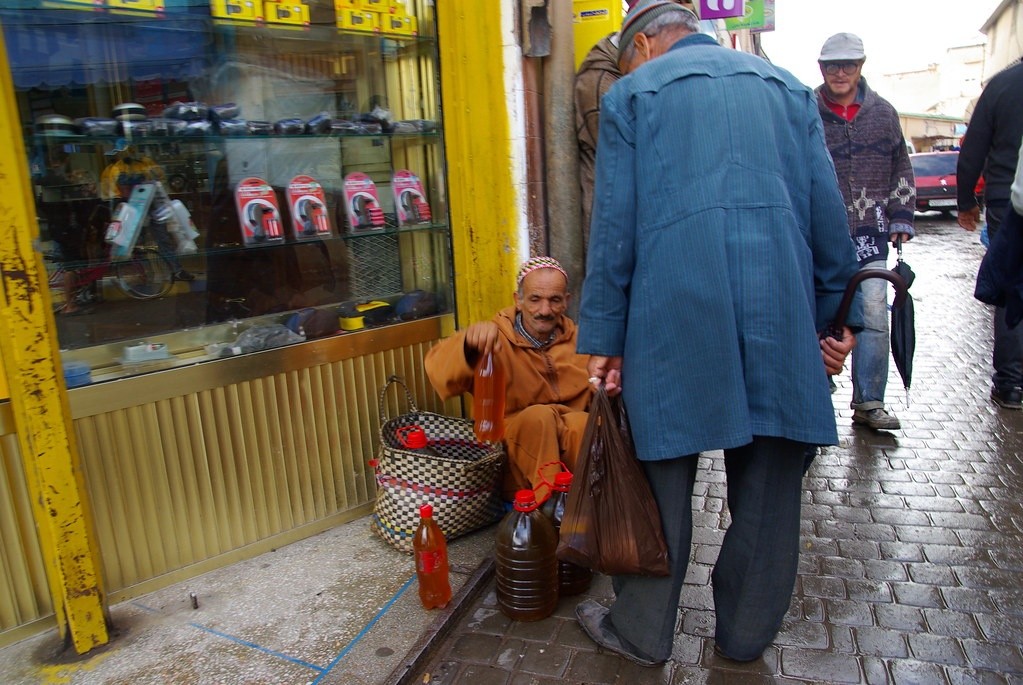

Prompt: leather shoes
xmin=576 ymin=598 xmax=666 ymax=669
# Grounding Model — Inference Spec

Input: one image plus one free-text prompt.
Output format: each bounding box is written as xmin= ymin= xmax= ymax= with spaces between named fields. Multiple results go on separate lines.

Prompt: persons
xmin=956 ymin=63 xmax=1023 ymax=408
xmin=575 ymin=32 xmax=625 ymax=258
xmin=426 ymin=256 xmax=585 ymax=505
xmin=814 ymin=33 xmax=917 ymax=429
xmin=576 ymin=0 xmax=866 ymax=663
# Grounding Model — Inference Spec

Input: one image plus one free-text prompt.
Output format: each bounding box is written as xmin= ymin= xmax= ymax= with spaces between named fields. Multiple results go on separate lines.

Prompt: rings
xmin=588 ymin=376 xmax=598 ymax=384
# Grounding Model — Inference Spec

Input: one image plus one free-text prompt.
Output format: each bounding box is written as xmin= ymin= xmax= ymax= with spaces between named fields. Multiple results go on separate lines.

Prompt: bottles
xmin=495 ymin=482 xmax=559 ymax=620
xmin=193 ymin=161 xmax=202 ymax=187
xmin=31 ymin=162 xmax=42 ymax=201
xmin=413 ymin=505 xmax=452 ymax=608
xmin=397 ymin=425 xmax=440 ymax=456
xmin=537 ymin=462 xmax=592 ymax=596
xmin=473 ymin=346 xmax=508 ymax=444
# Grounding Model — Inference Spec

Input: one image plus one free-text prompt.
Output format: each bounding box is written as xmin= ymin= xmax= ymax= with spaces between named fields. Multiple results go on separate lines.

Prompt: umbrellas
xmin=891 ymin=234 xmax=916 ymax=408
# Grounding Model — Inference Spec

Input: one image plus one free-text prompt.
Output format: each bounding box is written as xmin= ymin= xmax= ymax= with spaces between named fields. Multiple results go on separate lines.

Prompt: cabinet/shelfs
xmin=2 ymin=0 xmax=455 ymax=394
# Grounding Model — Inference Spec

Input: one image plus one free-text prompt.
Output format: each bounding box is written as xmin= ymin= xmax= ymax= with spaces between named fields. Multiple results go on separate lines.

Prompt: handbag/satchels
xmin=555 ymin=384 xmax=671 ymax=579
xmin=366 ymin=377 xmax=508 ymax=553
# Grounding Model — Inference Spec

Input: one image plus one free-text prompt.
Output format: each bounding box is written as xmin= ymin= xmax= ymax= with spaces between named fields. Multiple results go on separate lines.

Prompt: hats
xmin=818 ymin=33 xmax=865 ymax=60
xmin=617 ymin=0 xmax=697 ymax=70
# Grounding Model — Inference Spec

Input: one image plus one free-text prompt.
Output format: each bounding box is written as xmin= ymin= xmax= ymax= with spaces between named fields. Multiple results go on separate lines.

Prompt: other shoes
xmin=990 ymin=384 xmax=1023 ymax=408
xmin=851 ymin=406 xmax=900 ymax=430
xmin=826 ymin=373 xmax=837 ymax=392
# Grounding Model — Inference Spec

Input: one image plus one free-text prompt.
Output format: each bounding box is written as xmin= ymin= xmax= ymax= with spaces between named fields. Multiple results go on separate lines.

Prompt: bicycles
xmin=43 ymin=205 xmax=177 ymax=315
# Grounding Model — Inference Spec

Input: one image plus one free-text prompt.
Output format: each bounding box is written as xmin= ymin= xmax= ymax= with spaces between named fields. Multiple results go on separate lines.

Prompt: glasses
xmin=822 ymin=59 xmax=865 ymax=75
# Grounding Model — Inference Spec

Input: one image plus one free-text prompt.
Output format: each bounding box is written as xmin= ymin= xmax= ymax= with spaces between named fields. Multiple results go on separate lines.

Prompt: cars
xmin=906 ymin=150 xmax=987 ymax=216
